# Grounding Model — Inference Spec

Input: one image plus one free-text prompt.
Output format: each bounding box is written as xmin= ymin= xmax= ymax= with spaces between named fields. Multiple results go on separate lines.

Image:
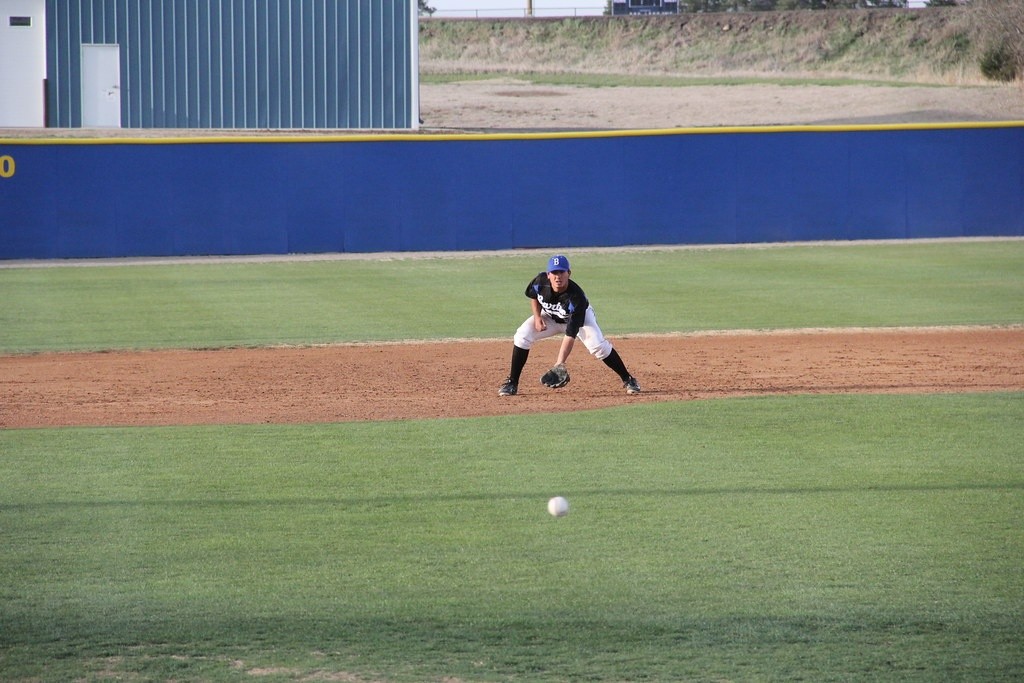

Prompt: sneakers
xmin=623 ymin=379 xmax=640 ymax=395
xmin=497 ymin=381 xmax=518 ymax=396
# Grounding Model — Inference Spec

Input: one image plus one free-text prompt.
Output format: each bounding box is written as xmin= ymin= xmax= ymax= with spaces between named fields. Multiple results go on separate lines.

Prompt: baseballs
xmin=548 ymin=496 xmax=568 ymax=518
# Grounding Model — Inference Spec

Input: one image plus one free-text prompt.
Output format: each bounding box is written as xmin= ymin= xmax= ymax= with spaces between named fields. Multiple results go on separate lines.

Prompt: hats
xmin=547 ymin=255 xmax=570 ymax=273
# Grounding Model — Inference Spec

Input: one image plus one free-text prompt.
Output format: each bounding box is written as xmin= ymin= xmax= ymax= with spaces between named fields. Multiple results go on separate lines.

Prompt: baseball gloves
xmin=539 ymin=362 xmax=570 ymax=388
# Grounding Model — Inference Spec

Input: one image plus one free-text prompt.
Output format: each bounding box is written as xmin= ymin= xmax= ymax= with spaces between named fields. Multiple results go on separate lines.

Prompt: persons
xmin=498 ymin=256 xmax=641 ymax=396
